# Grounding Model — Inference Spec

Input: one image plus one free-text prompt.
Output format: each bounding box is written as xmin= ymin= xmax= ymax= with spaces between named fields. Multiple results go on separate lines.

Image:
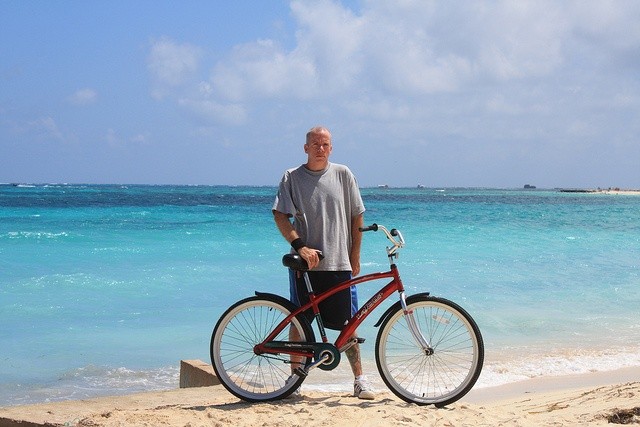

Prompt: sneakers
xmin=354 ymin=376 xmax=376 ymax=398
xmin=285 ymin=375 xmax=301 ymax=397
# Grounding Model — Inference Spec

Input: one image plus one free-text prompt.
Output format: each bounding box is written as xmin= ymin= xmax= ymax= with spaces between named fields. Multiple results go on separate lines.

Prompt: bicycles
xmin=211 ymin=224 xmax=483 ymax=406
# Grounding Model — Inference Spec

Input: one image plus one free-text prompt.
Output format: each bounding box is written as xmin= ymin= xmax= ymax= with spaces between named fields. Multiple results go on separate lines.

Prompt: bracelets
xmin=291 ymin=237 xmax=305 ymax=252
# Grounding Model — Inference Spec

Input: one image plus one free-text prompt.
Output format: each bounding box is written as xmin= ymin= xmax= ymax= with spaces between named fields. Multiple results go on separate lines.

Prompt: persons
xmin=272 ymin=126 xmax=375 ymax=399
